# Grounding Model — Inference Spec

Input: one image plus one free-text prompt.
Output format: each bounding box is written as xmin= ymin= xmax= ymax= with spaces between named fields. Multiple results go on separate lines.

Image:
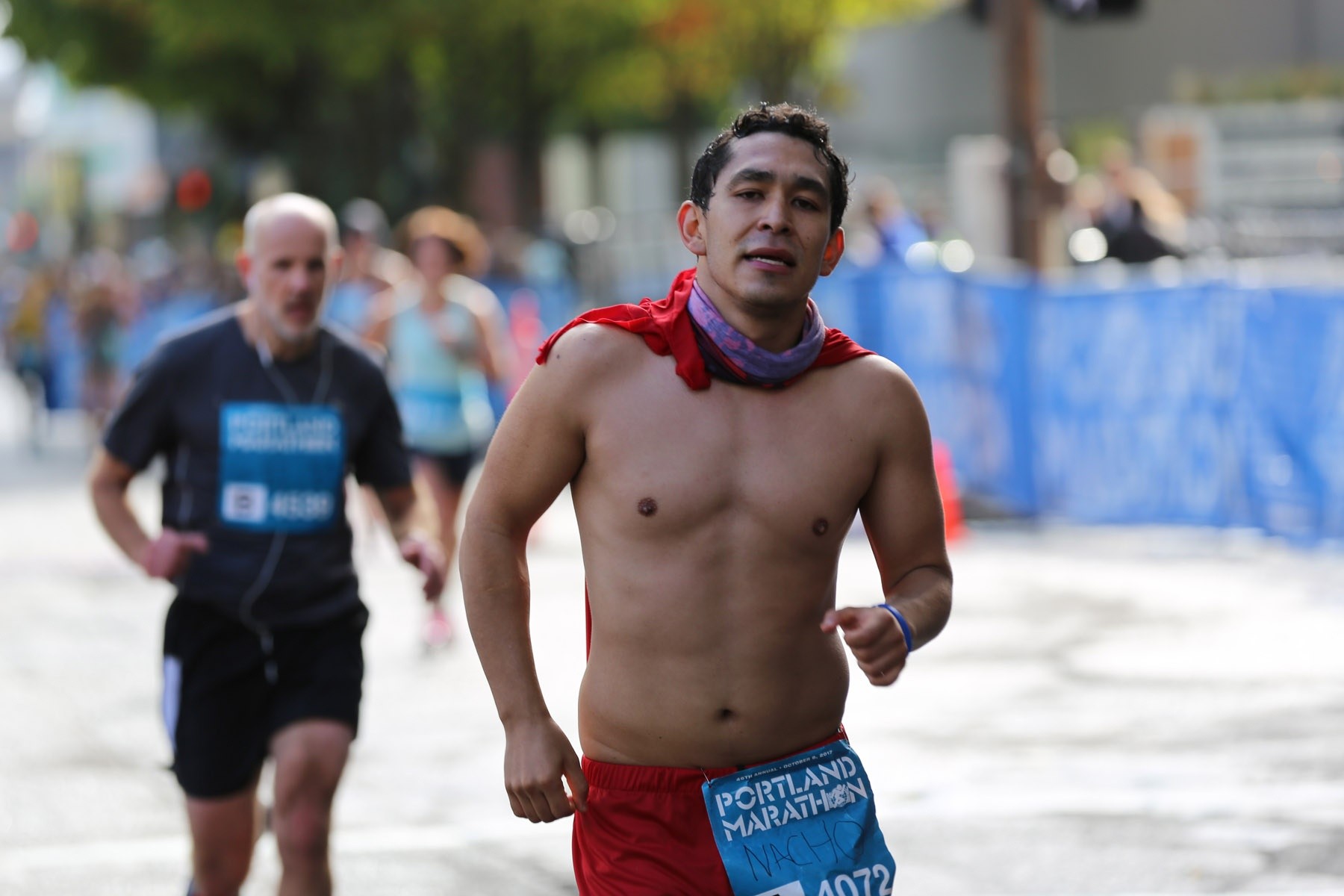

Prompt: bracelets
xmin=876 ymin=602 xmax=913 ymax=653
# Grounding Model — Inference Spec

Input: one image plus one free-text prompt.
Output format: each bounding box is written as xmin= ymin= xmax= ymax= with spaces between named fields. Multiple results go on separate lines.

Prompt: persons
xmin=459 ymin=101 xmax=952 ymax=896
xmin=1075 ymin=137 xmax=1185 ymax=264
xmin=3 ymin=104 xmax=952 ymax=896
